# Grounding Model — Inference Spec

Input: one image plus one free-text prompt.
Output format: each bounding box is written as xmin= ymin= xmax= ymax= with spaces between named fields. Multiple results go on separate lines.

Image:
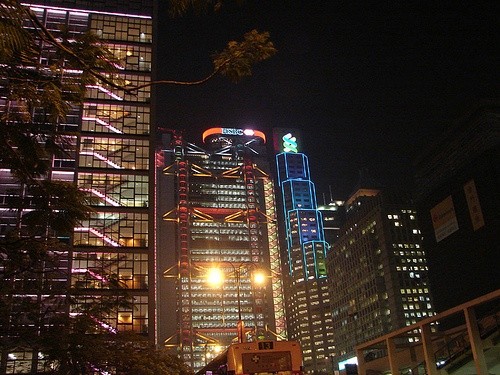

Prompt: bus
xmin=195 ymin=340 xmax=303 ymax=375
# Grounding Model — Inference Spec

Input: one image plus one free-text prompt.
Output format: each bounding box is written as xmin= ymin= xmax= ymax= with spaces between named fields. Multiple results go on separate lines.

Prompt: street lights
xmin=206 ymin=257 xmax=269 ymax=344
